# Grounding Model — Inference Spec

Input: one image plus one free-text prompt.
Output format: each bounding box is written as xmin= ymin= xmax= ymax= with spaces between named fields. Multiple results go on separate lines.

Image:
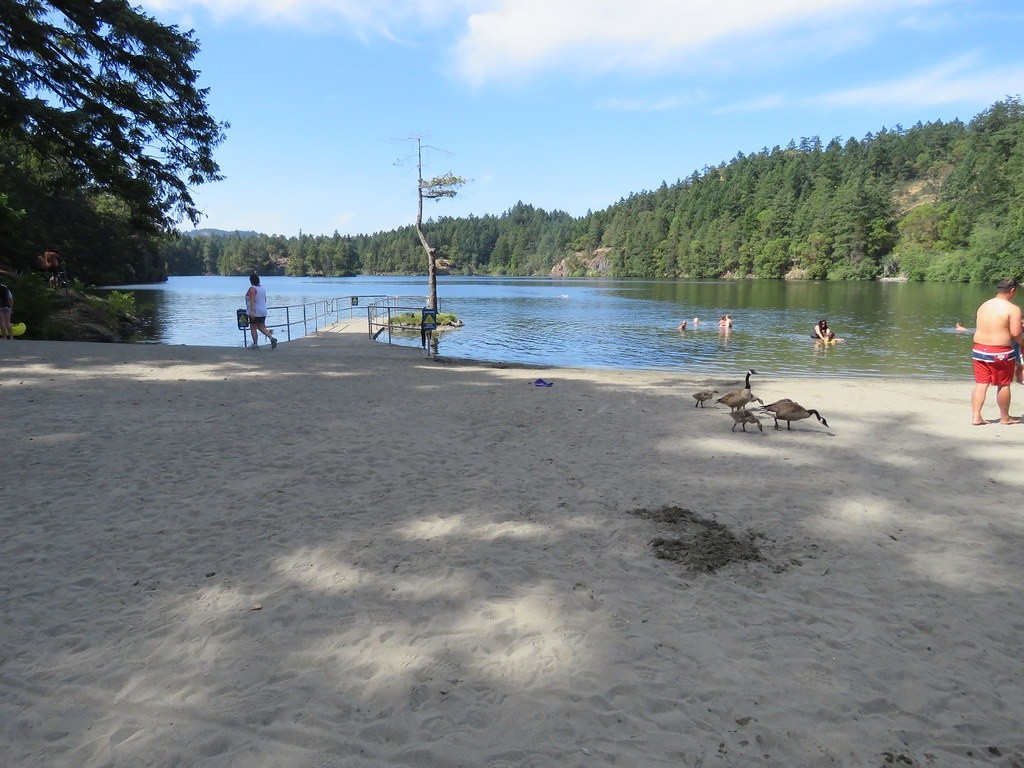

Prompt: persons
xmin=244 ymin=273 xmax=278 ymax=350
xmin=679 ymin=320 xmax=686 ymax=332
xmin=0 ymin=281 xmax=15 ymax=339
xmin=39 ymin=250 xmax=62 ymax=288
xmin=969 ymin=279 xmax=1024 ymax=424
xmin=810 ymin=319 xmax=830 ymax=340
xmin=692 ymin=317 xmax=701 ymax=324
xmin=719 ymin=314 xmax=733 ymax=328
xmin=956 ymin=320 xmax=966 ymax=330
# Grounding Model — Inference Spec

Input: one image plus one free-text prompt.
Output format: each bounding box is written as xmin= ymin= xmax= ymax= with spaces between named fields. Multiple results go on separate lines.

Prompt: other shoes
xmin=271 ymin=338 xmax=277 ymax=349
xmin=247 ymin=344 xmax=259 ymax=350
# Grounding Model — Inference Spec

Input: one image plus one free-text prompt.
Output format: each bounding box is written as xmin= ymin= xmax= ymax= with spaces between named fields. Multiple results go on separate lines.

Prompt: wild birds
xmin=729 ymin=410 xmax=763 ymax=432
xmin=715 ymin=369 xmax=758 ymax=411
xmin=759 ymin=398 xmax=829 ymax=430
xmin=739 ymin=394 xmax=763 ymax=410
xmin=692 ymin=389 xmax=719 ymax=408
